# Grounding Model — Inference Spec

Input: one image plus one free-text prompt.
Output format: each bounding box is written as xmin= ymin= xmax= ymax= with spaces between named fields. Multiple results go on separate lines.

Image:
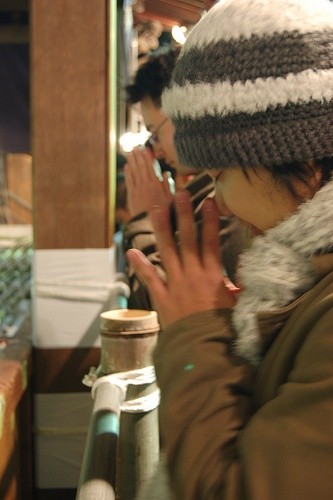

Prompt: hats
xmin=160 ymin=0 xmax=333 ymax=169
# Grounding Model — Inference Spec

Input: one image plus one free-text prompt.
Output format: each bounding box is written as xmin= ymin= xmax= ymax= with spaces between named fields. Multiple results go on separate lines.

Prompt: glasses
xmin=148 ymin=116 xmax=169 ymax=146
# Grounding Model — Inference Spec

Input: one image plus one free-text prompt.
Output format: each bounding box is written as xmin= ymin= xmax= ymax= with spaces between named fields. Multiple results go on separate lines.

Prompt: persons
xmin=127 ymin=0 xmax=333 ymax=500
xmin=112 ymin=43 xmax=254 ymax=313
